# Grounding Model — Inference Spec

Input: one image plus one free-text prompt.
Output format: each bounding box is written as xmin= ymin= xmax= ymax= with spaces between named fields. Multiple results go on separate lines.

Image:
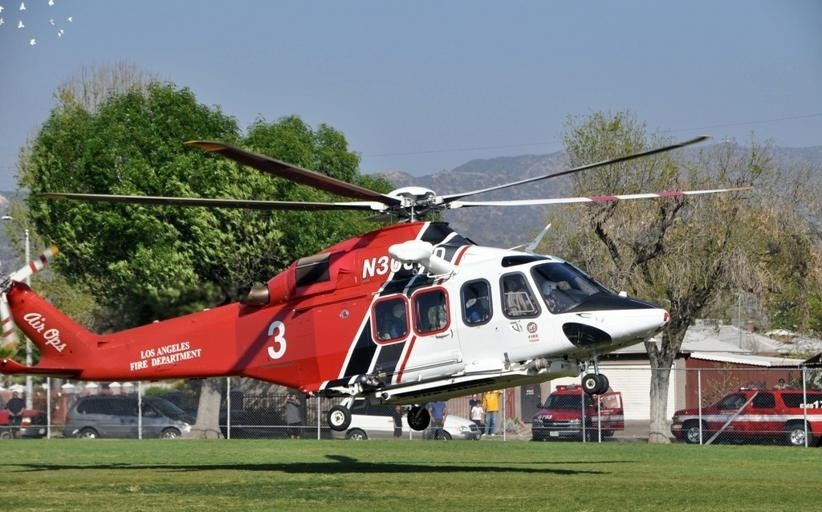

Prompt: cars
xmin=62 ymin=394 xmax=196 ymax=440
xmin=531 ymin=384 xmax=625 ymax=442
xmin=328 ymin=397 xmax=480 ymax=441
xmin=0 ymin=390 xmax=48 ymax=440
xmin=670 ymin=388 xmax=821 ymax=448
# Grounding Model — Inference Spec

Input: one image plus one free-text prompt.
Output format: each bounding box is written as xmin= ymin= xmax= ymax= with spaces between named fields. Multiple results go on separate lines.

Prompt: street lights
xmin=1 ymin=214 xmax=34 ymax=411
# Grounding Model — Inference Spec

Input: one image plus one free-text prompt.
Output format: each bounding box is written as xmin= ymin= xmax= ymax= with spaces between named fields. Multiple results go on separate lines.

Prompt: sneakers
xmin=481 ymin=434 xmax=496 ymax=436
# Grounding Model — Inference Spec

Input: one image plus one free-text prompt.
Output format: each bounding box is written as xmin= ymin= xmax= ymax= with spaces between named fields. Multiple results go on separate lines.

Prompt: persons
xmin=387 ymin=303 xmax=406 ymax=339
xmin=391 ymin=404 xmax=405 ymax=439
xmin=772 ymin=378 xmax=792 ymax=390
xmin=282 ymin=391 xmax=305 ymax=440
xmin=470 ymin=389 xmax=504 ymax=438
xmin=4 ymin=387 xmax=26 ymax=437
xmin=464 ymin=286 xmax=488 ymax=323
xmin=428 ymin=394 xmax=449 ymax=440
xmin=586 ymin=392 xmax=609 ymax=442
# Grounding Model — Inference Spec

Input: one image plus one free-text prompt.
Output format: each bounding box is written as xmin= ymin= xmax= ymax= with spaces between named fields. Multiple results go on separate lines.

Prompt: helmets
xmin=391 ymin=303 xmax=406 ymax=318
xmin=542 ymin=280 xmax=558 ymax=296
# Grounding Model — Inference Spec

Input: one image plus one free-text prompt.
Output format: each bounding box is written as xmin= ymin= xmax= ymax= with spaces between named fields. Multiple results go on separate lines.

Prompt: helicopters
xmin=1 ymin=136 xmax=754 ymax=431
